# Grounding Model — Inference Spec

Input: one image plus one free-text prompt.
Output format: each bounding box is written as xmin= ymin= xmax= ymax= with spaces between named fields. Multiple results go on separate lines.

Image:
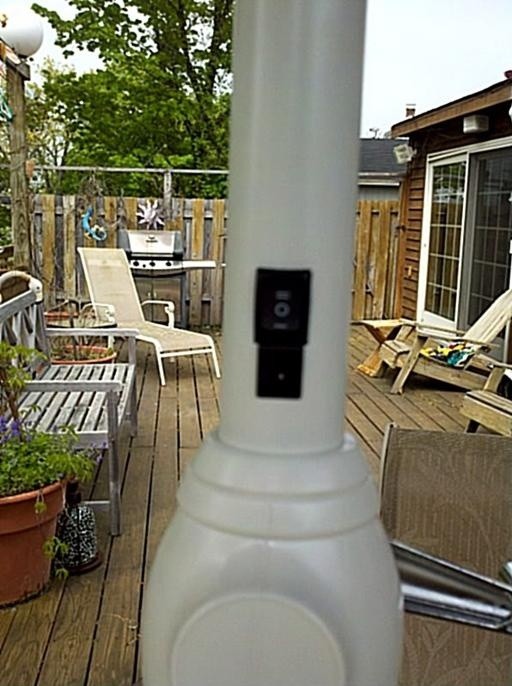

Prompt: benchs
xmin=0 ymin=289 xmax=143 ymax=535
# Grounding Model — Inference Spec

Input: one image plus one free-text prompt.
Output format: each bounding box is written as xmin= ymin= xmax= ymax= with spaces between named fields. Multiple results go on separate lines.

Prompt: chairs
xmin=348 ymin=289 xmax=512 ymax=398
xmin=72 ymin=241 xmax=220 ymax=390
xmin=459 ymin=360 xmax=512 ymax=438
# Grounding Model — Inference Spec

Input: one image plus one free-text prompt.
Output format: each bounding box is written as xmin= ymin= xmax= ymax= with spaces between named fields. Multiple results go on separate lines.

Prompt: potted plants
xmin=1 ymin=339 xmax=118 ymax=606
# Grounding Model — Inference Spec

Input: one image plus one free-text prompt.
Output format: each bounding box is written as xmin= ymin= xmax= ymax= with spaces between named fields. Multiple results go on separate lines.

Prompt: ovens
xmin=118 ymin=228 xmax=184 ymax=259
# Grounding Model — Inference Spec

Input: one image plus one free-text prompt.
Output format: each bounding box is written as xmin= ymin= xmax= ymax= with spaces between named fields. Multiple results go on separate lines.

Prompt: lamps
xmin=392 ymin=143 xmax=422 ymax=164
